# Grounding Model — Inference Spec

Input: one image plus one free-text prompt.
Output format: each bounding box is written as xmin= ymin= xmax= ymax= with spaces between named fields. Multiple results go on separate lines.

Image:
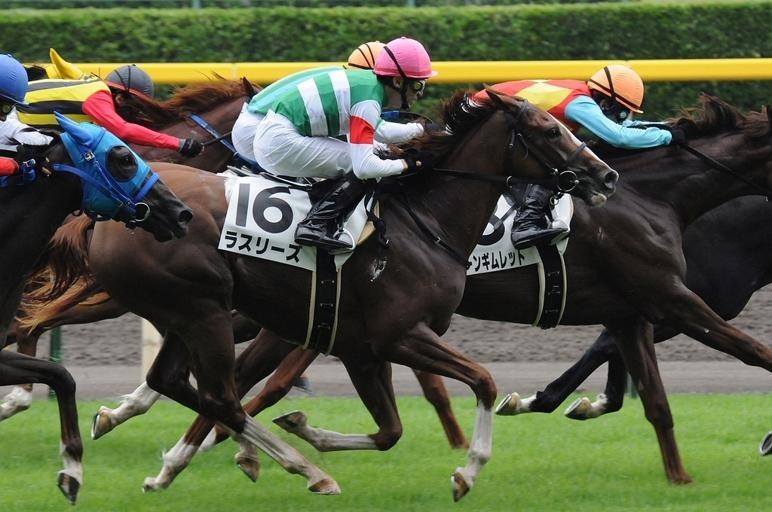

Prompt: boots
xmin=511 ymin=187 xmax=567 ymax=250
xmin=294 ymin=179 xmax=369 ymax=249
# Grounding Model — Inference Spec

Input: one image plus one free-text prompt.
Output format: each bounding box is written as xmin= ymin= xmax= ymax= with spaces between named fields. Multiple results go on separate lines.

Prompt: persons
xmin=253 ymin=35 xmax=433 ymax=250
xmin=233 ymin=40 xmax=403 ymax=162
xmin=18 ymin=65 xmax=204 ymax=158
xmin=446 ymin=64 xmax=685 ymax=250
xmin=0 ymin=53 xmax=52 ymax=175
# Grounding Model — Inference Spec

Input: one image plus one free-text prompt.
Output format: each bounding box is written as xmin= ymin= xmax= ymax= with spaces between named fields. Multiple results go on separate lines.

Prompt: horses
xmin=0 ymin=71 xmax=772 ymax=506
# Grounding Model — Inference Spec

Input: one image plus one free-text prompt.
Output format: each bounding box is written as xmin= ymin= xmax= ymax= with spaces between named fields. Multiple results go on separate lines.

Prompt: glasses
xmin=615 ymin=108 xmax=627 ymax=122
xmin=0 ymin=101 xmax=14 ymax=117
xmin=405 ymin=82 xmax=424 ymax=92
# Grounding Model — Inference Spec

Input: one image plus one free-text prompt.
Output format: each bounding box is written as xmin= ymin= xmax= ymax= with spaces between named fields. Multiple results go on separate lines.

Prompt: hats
xmin=104 ymin=64 xmax=154 ymax=103
xmin=0 ymin=54 xmax=29 ymax=105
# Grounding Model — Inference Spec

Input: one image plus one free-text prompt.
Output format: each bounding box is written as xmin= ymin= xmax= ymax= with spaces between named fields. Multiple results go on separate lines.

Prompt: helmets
xmin=344 ymin=38 xmax=438 ymax=78
xmin=587 ymin=65 xmax=644 ymax=115
